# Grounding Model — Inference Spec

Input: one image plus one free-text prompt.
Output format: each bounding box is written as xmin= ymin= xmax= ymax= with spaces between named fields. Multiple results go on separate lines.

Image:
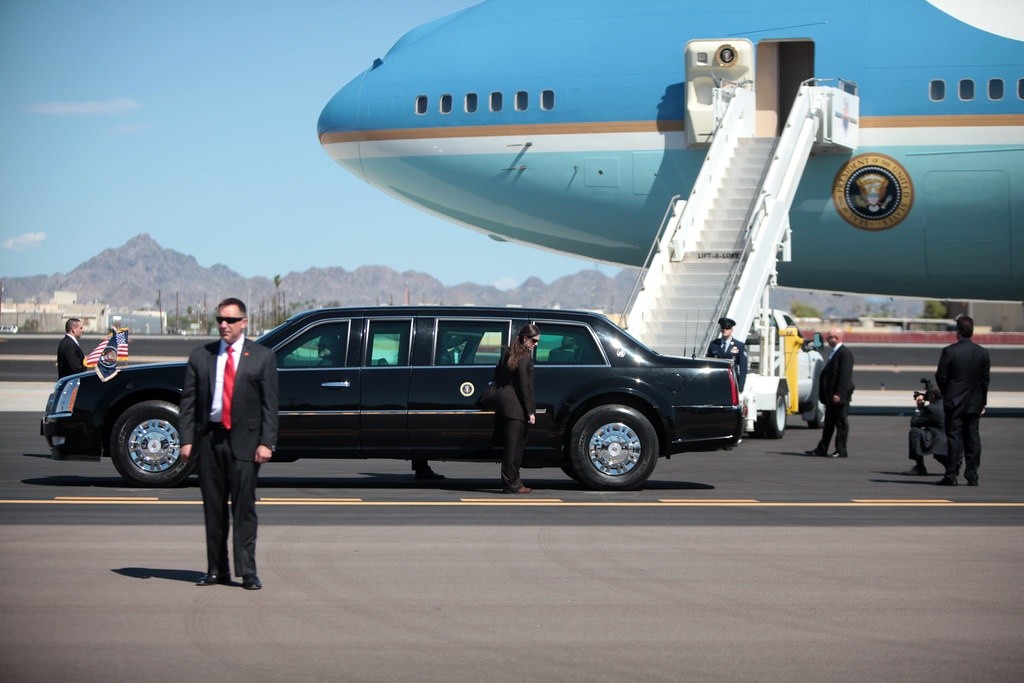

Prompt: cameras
xmin=914 ymin=378 xmax=929 ymax=401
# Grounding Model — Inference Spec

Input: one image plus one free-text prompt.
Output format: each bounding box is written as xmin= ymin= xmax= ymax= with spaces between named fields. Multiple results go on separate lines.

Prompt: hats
xmin=717 ymin=318 xmax=736 ymax=329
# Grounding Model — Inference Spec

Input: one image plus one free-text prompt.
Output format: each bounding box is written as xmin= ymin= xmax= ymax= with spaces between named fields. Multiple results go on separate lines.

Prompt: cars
xmin=39 ymin=305 xmax=744 ymax=490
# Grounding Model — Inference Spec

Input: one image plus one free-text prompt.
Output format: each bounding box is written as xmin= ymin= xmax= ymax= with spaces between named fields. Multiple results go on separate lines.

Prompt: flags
xmin=83 ymin=327 xmax=128 ymax=367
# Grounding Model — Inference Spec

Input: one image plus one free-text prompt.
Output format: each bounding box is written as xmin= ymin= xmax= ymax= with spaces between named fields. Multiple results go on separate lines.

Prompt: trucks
xmin=740 ymin=309 xmax=825 ymax=440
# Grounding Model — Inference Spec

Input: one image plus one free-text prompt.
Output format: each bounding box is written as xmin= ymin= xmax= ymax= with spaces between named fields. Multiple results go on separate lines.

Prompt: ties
xmin=221 ymin=347 xmax=235 ymax=430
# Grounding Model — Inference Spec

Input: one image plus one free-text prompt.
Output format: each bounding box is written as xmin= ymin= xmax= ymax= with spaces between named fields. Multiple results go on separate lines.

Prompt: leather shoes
xmin=966 ymin=481 xmax=978 ymax=486
xmin=903 ymin=465 xmax=928 ymax=476
xmin=195 ymin=574 xmax=232 ymax=585
xmin=518 ymin=487 xmax=531 ymax=493
xmin=242 ymin=575 xmax=261 ymax=590
xmin=828 ymin=452 xmax=848 ymax=458
xmin=414 ymin=468 xmax=446 ymax=480
xmin=935 ymin=476 xmax=958 ymax=486
xmin=804 ymin=449 xmax=828 ymax=457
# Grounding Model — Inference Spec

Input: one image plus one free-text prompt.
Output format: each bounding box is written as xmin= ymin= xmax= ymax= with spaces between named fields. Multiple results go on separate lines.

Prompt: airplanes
xmin=316 ymin=0 xmax=1024 ymax=304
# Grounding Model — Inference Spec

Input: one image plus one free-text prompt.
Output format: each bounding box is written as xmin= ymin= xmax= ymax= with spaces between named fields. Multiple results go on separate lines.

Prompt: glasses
xmin=528 ymin=337 xmax=539 ymax=342
xmin=215 ymin=316 xmax=245 ymax=325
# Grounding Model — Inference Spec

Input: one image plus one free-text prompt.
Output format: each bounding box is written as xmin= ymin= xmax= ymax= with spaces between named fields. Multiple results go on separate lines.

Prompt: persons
xmin=935 ymin=316 xmax=991 ymax=486
xmin=804 ymin=327 xmax=856 ymax=458
xmin=902 ymin=386 xmax=960 ymax=477
xmin=447 ymin=341 xmax=467 ymax=365
xmin=57 ymin=318 xmax=87 ymax=380
xmin=412 ymin=460 xmax=445 ymax=480
xmin=177 ymin=297 xmax=279 ymax=589
xmin=705 ymin=317 xmax=748 ymax=393
xmin=548 ymin=336 xmax=579 ymax=365
xmin=493 ymin=324 xmax=541 ymax=494
